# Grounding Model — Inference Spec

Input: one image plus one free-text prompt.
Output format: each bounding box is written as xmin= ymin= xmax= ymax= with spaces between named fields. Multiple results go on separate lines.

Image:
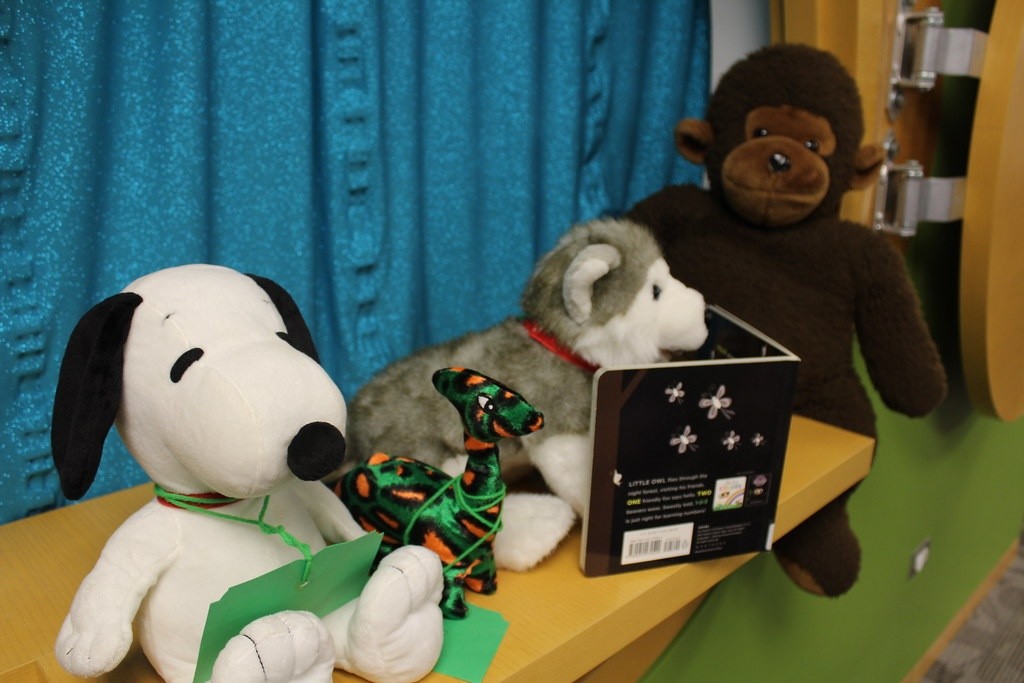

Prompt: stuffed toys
xmin=345 ymin=218 xmax=708 ymax=573
xmin=51 ymin=263 xmax=445 ymax=683
xmin=614 ymin=42 xmax=947 ymax=598
xmin=334 ymin=366 xmax=545 ymax=619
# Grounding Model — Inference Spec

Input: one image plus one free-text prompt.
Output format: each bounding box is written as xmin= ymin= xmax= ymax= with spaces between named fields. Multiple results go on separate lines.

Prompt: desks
xmin=0 ymin=386 xmax=873 ymax=682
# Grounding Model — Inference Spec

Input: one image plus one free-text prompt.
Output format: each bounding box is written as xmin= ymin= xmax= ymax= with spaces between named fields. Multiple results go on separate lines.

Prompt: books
xmin=580 ymin=306 xmax=802 ymax=576
